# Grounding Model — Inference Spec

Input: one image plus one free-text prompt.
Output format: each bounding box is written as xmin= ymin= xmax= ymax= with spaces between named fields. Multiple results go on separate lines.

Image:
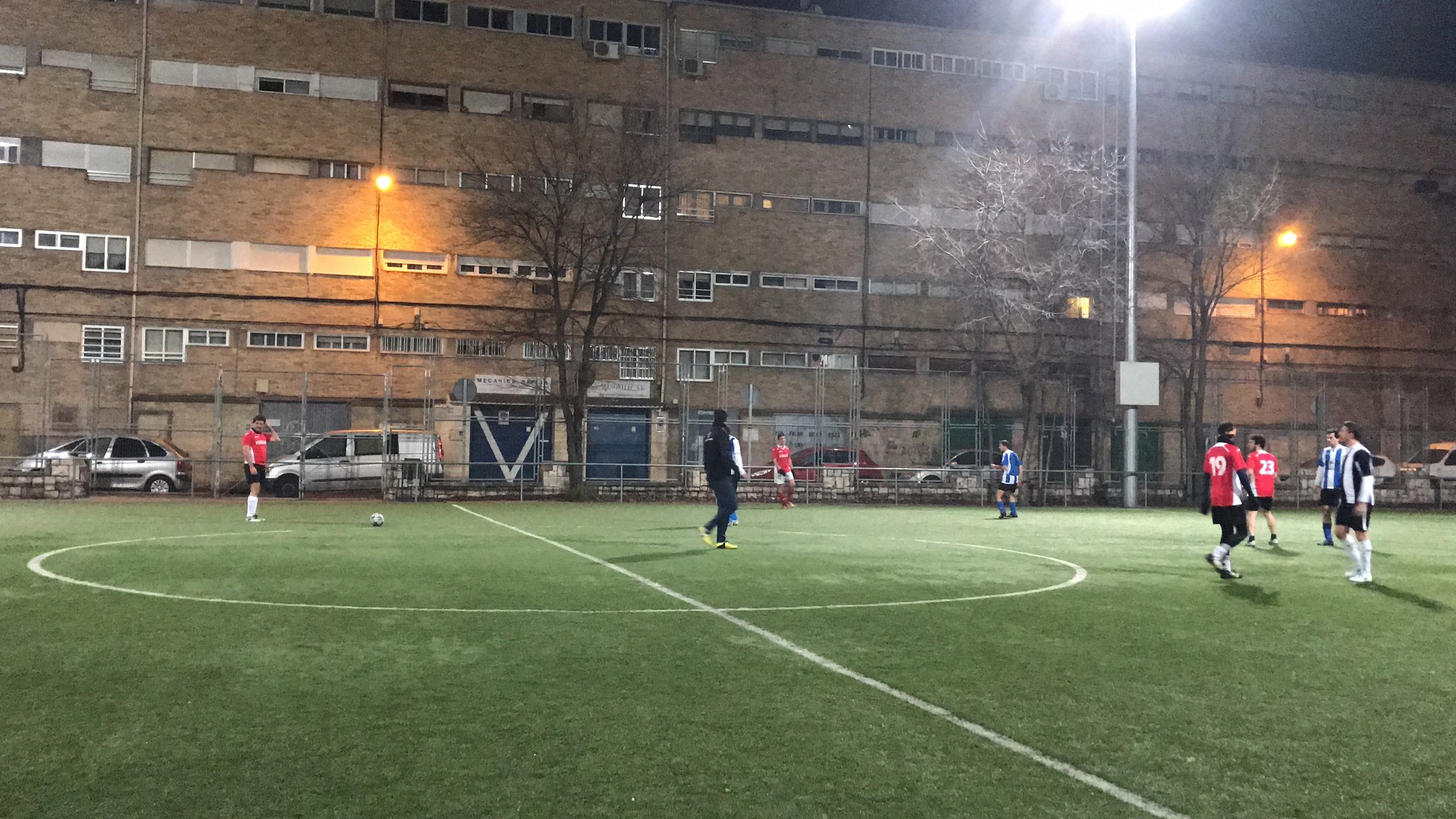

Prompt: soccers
xmin=369 ymin=512 xmax=385 ymax=527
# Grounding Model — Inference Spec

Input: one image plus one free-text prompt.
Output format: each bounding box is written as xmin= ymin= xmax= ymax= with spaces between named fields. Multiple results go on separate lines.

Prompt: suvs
xmin=262 ymin=429 xmax=447 ymax=497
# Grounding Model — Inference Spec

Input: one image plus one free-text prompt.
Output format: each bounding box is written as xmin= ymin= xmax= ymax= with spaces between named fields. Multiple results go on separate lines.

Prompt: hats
xmin=714 ymin=409 xmax=728 ymax=422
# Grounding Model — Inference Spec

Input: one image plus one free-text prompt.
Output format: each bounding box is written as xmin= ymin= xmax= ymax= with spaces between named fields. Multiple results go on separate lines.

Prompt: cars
xmin=898 ymin=448 xmax=1004 ymax=488
xmin=8 ymin=435 xmax=193 ymax=492
xmin=742 ymin=446 xmax=885 ymax=482
xmin=1273 ymin=452 xmax=1397 ymax=491
xmin=1397 ymin=442 xmax=1456 ymax=481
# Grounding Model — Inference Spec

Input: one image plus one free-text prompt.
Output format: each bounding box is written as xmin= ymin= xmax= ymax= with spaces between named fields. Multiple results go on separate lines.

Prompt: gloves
xmin=733 ymin=465 xmax=740 ymax=481
xmin=791 ymin=469 xmax=795 ymax=476
xmin=779 ymin=469 xmax=785 ymax=477
xmin=1201 ymin=498 xmax=1210 ymax=514
xmin=1247 ymin=496 xmax=1256 ymax=508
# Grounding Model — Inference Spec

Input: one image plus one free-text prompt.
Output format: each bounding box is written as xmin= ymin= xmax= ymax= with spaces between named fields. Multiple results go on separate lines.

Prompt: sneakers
xmin=716 ymin=542 xmax=738 ymax=548
xmin=999 ymin=512 xmax=1007 ymax=519
xmin=1008 ymin=512 xmax=1017 ymax=518
xmin=699 ymin=526 xmax=713 ymax=546
xmin=1246 ymin=539 xmax=1256 ymax=547
xmin=1269 ymin=539 xmax=1278 ymax=545
xmin=1350 ymin=573 xmax=1372 ymax=583
xmin=1344 ymin=568 xmax=1361 ymax=578
xmin=1220 ymin=569 xmax=1242 ymax=579
xmin=246 ymin=515 xmax=266 ymax=522
xmin=1206 ymin=554 xmax=1226 ymax=575
xmin=1317 ymin=540 xmax=1334 ymax=546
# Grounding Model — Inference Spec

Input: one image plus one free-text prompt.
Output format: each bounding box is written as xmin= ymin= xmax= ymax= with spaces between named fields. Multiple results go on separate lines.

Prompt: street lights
xmin=1084 ymin=0 xmax=1183 ymax=508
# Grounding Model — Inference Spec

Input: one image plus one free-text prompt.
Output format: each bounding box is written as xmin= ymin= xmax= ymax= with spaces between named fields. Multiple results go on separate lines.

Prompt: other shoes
xmin=728 ymin=518 xmax=738 ymax=527
xmin=788 ymin=501 xmax=795 ymax=508
xmin=782 ymin=503 xmax=787 ymax=509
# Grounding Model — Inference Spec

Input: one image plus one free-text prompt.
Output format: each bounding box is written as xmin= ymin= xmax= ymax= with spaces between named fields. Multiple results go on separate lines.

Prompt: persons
xmin=1200 ymin=421 xmax=1375 ymax=584
xmin=990 ymin=440 xmax=1023 ymax=519
xmin=698 ymin=409 xmax=751 ymax=550
xmin=771 ymin=433 xmax=797 ymax=509
xmin=242 ymin=416 xmax=281 ymax=522
